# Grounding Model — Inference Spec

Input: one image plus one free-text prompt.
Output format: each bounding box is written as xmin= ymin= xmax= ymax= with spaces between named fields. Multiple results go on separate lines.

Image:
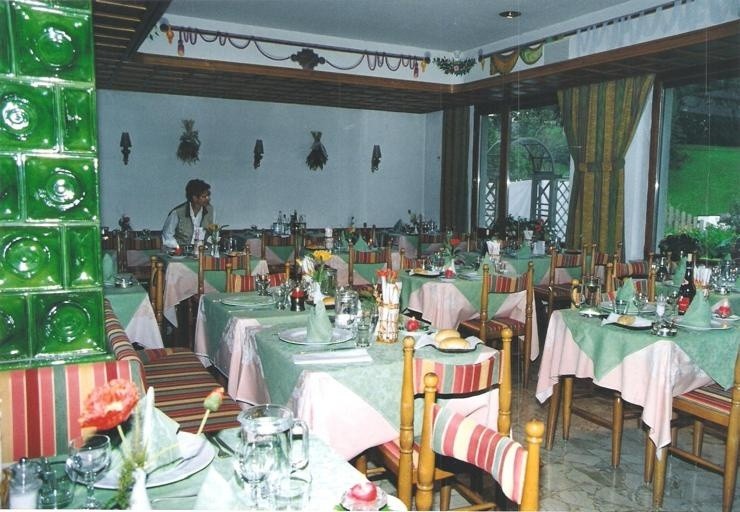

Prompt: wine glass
xmin=569 ymin=249 xmax=740 ymax=338
xmin=67 ymin=434 xmax=112 ymax=510
xmin=412 ymin=248 xmax=507 ymax=279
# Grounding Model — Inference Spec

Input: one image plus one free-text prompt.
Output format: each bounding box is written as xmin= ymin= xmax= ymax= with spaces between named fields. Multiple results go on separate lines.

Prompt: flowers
xmin=76 ymin=378 xmax=146 ymax=511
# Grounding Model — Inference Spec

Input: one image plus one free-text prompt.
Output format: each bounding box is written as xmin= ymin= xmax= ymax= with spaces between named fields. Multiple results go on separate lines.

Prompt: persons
xmin=161 ymin=179 xmax=216 ymax=256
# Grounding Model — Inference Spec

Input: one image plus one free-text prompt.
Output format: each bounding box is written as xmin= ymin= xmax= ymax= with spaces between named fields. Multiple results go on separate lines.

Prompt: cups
xmin=101 ymin=224 xmax=151 ymax=244
xmin=166 ymin=236 xmax=248 ymax=257
xmin=256 ymin=268 xmax=380 ymax=349
xmin=34 ymin=457 xmax=80 ymax=509
xmin=233 ymin=403 xmax=312 ymax=511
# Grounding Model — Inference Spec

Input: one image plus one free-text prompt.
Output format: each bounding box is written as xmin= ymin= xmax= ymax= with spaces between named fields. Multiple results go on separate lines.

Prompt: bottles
xmin=324 ymin=215 xmax=379 ymax=250
xmin=481 ymin=226 xmax=566 ymax=256
xmin=273 ymin=210 xmax=307 ymax=238
xmin=399 ymin=209 xmax=452 ymax=234
xmin=2 ymin=453 xmax=43 ymax=509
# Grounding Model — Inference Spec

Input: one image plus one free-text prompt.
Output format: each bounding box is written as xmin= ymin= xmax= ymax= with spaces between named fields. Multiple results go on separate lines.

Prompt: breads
xmin=440 ymin=337 xmax=470 ymax=351
xmin=435 ymin=329 xmax=460 ymax=343
xmin=617 ymin=314 xmax=635 ymax=325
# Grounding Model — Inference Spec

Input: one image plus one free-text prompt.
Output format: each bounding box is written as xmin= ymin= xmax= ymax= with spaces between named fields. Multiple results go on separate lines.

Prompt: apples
xmin=719 ymin=306 xmax=730 ymax=317
xmin=407 ymin=321 xmax=419 ymax=330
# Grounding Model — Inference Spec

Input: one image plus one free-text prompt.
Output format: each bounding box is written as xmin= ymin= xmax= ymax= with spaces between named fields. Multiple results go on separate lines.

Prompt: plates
xmin=103 ymin=270 xmax=137 ymax=289
xmin=396 ymin=318 xmax=432 ymax=336
xmin=66 ymin=431 xmax=217 ymax=491
xmin=221 ymin=294 xmax=277 ymax=307
xmin=426 ymin=331 xmax=478 ymax=354
